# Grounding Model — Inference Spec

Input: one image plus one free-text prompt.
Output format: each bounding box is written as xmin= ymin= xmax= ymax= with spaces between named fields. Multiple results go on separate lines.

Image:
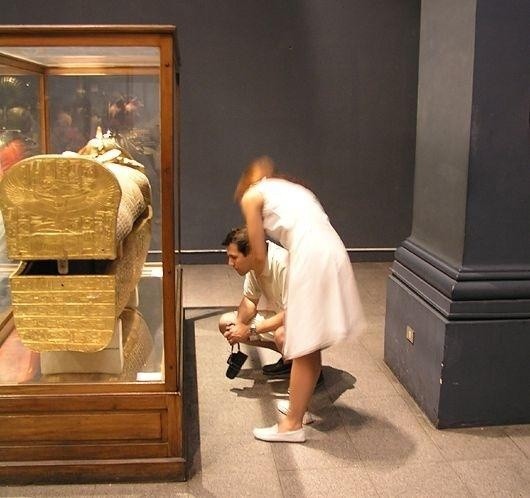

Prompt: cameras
xmin=227 ymin=352 xmax=248 ymax=378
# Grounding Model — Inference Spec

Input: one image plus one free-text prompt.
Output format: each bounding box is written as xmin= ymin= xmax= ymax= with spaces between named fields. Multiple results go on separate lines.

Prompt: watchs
xmin=247 ymin=323 xmax=257 ymax=335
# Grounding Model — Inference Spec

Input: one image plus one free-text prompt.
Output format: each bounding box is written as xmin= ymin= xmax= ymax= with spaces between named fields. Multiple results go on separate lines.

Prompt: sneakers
xmin=288 ymin=370 xmax=324 ymax=394
xmin=253 ymin=422 xmax=306 ymax=443
xmin=262 ymin=357 xmax=292 ymax=374
xmin=278 ymin=399 xmax=313 ymax=425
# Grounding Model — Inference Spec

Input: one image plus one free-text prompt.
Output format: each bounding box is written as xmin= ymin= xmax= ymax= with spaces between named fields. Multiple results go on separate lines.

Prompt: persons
xmin=219 ymin=222 xmax=325 ymax=394
xmin=230 ymin=155 xmax=366 ymax=445
